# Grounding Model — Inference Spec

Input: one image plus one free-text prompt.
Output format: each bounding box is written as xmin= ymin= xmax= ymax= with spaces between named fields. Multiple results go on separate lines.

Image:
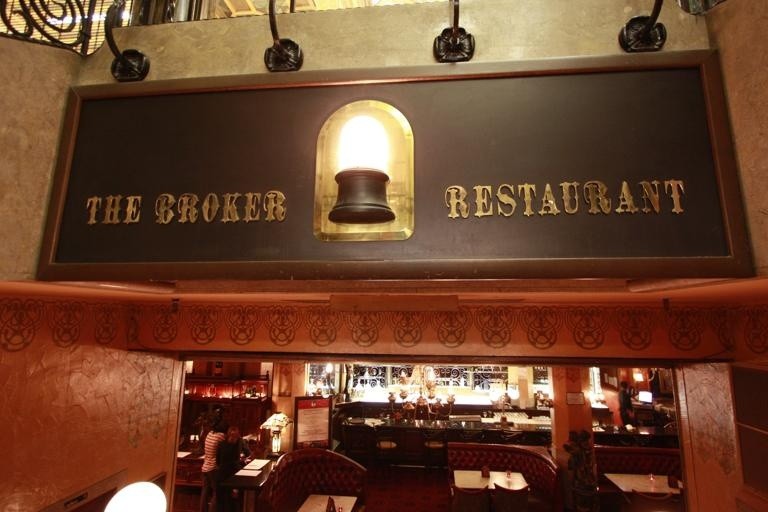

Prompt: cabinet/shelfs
xmin=463 ymin=399 xmax=668 ymax=444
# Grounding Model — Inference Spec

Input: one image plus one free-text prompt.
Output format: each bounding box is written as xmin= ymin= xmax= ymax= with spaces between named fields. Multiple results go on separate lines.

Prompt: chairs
xmin=576 ymin=439 xmax=689 ymax=507
xmin=362 ymin=423 xmax=454 ymax=470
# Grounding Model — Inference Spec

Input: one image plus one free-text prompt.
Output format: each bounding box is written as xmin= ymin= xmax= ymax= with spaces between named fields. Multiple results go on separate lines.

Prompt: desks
xmin=176 ymin=443 xmax=209 ymax=491
xmin=451 ymin=464 xmax=529 ymax=505
xmin=295 ymin=490 xmax=358 ymax=512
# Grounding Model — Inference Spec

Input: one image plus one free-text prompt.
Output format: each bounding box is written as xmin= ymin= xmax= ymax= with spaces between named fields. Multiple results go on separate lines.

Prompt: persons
xmin=618 ymin=380 xmax=635 ymax=425
xmin=199 ymin=423 xmax=227 ymax=512
xmin=213 ymin=404 xmax=229 ymax=432
xmin=216 ymin=425 xmax=251 ymax=512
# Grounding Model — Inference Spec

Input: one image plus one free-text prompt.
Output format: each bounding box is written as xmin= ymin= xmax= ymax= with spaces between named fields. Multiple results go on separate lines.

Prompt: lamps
xmin=377 ymin=367 xmax=457 ymax=426
